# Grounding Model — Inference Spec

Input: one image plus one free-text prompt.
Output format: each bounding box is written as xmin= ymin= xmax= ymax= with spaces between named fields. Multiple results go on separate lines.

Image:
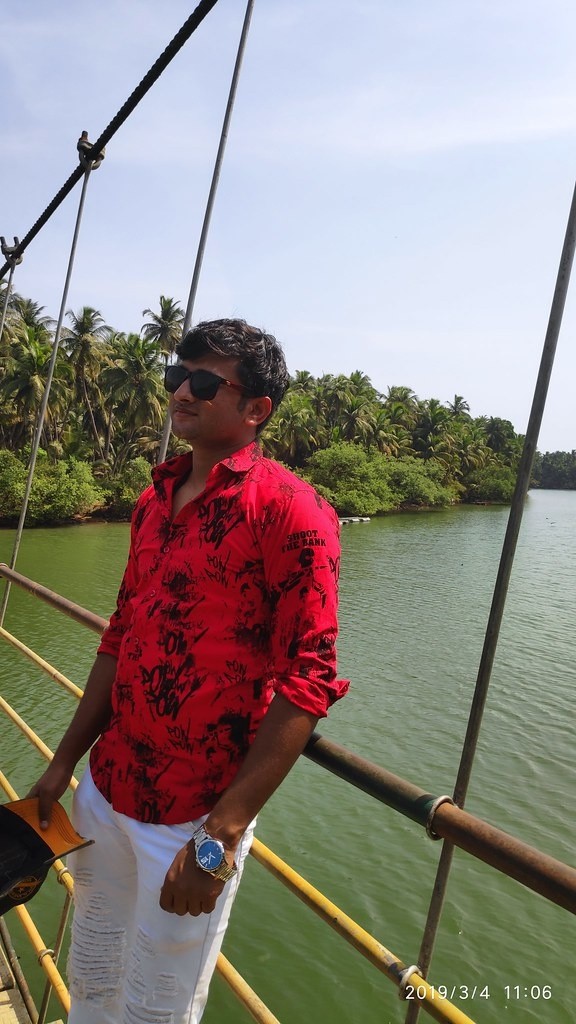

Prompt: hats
xmin=0 ymin=797 xmax=96 ymax=916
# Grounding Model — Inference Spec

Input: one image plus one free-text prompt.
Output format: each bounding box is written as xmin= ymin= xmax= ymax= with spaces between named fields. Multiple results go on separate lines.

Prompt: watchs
xmin=193 ymin=823 xmax=237 ymax=882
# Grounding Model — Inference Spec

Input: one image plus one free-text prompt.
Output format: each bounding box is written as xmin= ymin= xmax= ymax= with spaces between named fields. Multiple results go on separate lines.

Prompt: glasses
xmin=164 ymin=365 xmax=251 ymax=401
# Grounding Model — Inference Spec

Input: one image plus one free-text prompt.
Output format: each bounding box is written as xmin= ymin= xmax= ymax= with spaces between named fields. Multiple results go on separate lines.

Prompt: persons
xmin=25 ymin=320 xmax=350 ymax=1024
